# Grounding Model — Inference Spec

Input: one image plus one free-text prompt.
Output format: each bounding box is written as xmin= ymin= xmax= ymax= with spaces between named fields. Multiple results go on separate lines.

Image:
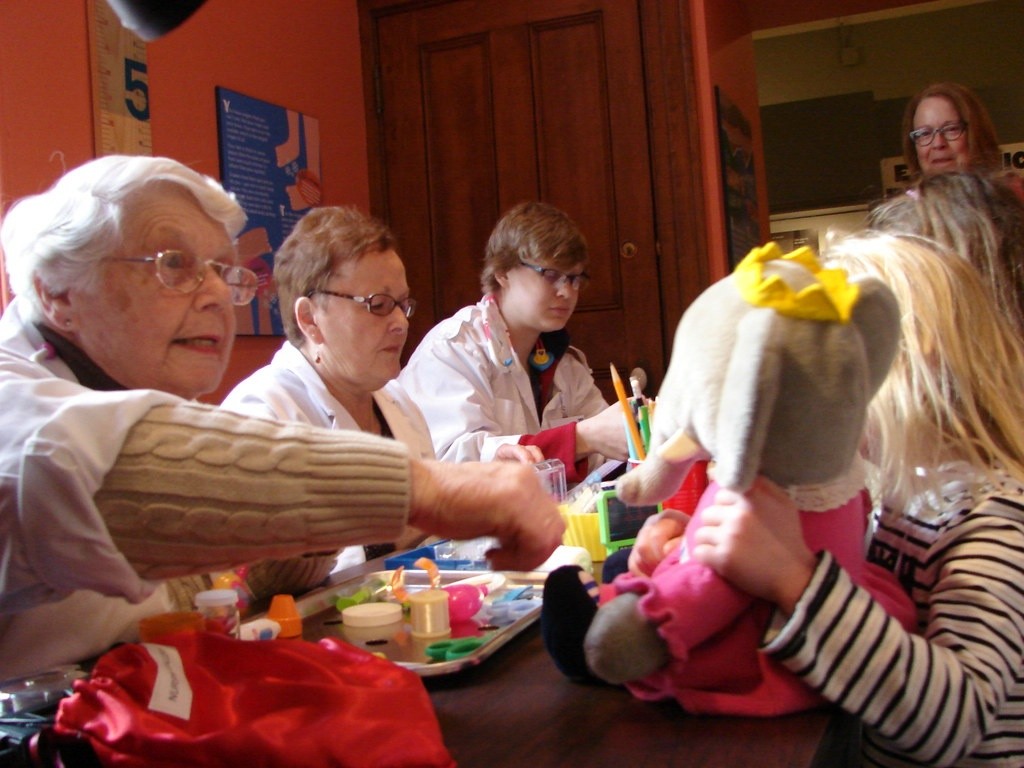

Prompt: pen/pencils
xmin=610 ymin=362 xmax=657 ymax=462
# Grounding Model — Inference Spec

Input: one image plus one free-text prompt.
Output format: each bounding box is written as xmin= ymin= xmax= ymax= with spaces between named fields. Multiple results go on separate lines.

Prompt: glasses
xmin=99 ymin=248 xmax=259 ymax=306
xmin=908 ymin=122 xmax=965 ymax=146
xmin=519 ymin=261 xmax=591 ymax=292
xmin=304 ymin=289 xmax=417 ymax=318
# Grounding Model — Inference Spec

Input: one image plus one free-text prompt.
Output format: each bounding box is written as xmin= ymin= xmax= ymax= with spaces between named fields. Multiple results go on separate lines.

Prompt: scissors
xmin=425 ymin=627 xmax=503 ymax=662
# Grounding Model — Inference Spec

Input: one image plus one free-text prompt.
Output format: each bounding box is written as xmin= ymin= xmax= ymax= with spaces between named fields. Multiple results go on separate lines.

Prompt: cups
xmin=627 ymin=458 xmax=710 ymax=518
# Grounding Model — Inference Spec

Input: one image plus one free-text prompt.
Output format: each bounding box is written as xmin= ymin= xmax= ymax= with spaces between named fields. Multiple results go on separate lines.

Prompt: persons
xmin=400 ymin=201 xmax=642 ymax=485
xmin=902 ymin=82 xmax=1024 ymax=204
xmin=628 ymin=231 xmax=1024 ymax=768
xmin=872 ymin=166 xmax=1024 ymax=336
xmin=0 ymin=156 xmax=565 ymax=680
xmin=220 ymin=206 xmax=545 ymax=575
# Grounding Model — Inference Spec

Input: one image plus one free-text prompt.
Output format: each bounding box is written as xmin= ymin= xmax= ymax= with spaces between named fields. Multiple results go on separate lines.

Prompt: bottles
xmin=193 ymin=589 xmax=241 ymax=640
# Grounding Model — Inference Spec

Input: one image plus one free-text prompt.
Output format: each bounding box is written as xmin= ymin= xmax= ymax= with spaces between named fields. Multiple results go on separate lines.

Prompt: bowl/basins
xmin=555 ymin=512 xmax=609 ymax=562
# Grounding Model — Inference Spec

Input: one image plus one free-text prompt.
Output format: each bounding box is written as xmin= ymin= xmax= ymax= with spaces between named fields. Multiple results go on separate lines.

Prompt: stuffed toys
xmin=540 ymin=244 xmax=913 ymax=717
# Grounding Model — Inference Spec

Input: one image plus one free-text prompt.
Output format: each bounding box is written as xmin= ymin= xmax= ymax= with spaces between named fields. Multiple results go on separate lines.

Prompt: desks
xmin=243 ymin=548 xmax=834 ymax=768
